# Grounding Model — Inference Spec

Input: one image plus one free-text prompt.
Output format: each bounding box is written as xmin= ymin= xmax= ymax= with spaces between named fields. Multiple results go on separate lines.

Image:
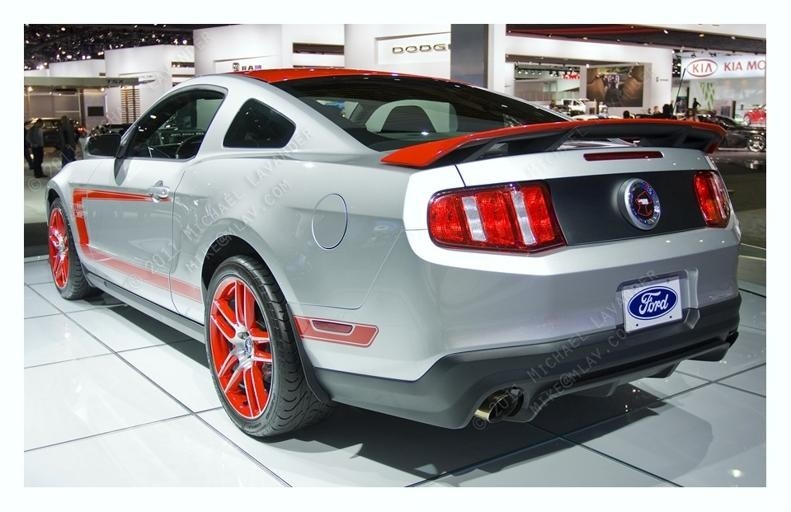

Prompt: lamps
xmin=517 ymin=68 xmax=580 ymax=79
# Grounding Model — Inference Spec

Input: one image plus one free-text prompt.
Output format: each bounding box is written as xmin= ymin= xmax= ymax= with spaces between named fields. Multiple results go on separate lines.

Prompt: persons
xmin=24 ymin=115 xmax=76 ymax=178
xmin=549 ymin=96 xmax=701 ymax=120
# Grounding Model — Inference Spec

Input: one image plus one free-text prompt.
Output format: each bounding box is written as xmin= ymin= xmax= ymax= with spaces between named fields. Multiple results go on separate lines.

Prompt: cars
xmin=681 ymin=113 xmax=765 ymax=155
xmin=25 ymin=114 xmax=87 ymax=147
xmin=556 ymin=95 xmax=609 ymax=115
xmin=81 ymin=121 xmax=133 ymax=143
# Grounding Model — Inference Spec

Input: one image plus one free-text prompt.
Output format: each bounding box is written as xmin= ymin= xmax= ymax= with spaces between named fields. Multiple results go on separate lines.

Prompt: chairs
xmin=381 ymin=105 xmax=438 ymax=133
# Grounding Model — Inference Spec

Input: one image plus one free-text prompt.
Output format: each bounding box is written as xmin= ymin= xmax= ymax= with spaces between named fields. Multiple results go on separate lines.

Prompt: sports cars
xmin=48 ymin=67 xmax=748 ymax=447
